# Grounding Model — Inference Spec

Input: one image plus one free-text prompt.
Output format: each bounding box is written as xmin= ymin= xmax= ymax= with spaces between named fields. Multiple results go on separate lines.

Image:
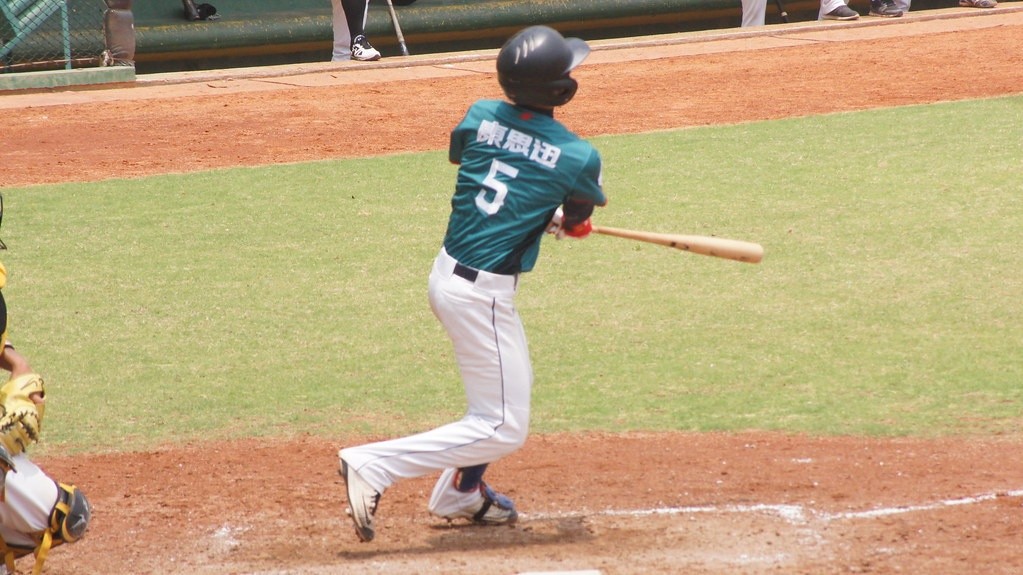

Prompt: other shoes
xmin=959 ymin=0 xmax=997 ymax=7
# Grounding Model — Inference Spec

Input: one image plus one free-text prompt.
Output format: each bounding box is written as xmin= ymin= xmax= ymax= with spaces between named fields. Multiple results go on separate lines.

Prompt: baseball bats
xmin=593 ymin=227 xmax=763 ymax=264
xmin=774 ymin=0 xmax=789 ymax=23
xmin=387 ymin=0 xmax=409 ymax=56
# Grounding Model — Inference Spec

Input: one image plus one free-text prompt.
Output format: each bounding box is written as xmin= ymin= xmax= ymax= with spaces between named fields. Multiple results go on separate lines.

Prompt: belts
xmin=453 ymin=263 xmax=479 ymax=282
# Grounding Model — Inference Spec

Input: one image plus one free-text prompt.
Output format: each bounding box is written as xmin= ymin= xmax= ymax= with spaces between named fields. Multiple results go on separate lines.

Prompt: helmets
xmin=496 ymin=25 xmax=591 ymax=106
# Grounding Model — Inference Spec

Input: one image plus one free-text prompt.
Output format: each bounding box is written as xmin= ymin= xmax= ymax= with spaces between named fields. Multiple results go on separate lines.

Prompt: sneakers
xmin=428 ymin=481 xmax=518 ymax=525
xmin=869 ymin=0 xmax=903 ymax=17
xmin=339 ymin=456 xmax=380 ymax=543
xmin=350 ymin=32 xmax=381 ymax=61
xmin=822 ymin=5 xmax=860 ymax=20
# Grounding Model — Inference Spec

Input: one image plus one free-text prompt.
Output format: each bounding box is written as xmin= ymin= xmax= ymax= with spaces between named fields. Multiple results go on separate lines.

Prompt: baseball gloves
xmin=0 ymin=374 xmax=46 ymax=456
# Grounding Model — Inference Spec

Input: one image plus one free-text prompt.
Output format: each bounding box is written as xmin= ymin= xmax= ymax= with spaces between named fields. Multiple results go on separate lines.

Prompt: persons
xmin=0 ymin=192 xmax=91 ymax=575
xmin=741 ymin=0 xmax=998 ymax=27
xmin=336 ymin=25 xmax=607 ymax=544
xmin=330 ymin=0 xmax=382 ymax=61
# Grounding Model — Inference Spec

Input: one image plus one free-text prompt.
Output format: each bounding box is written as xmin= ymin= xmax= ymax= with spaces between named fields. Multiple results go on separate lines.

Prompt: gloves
xmin=563 ymin=216 xmax=593 ymax=238
xmin=545 ymin=207 xmax=564 ymax=240
